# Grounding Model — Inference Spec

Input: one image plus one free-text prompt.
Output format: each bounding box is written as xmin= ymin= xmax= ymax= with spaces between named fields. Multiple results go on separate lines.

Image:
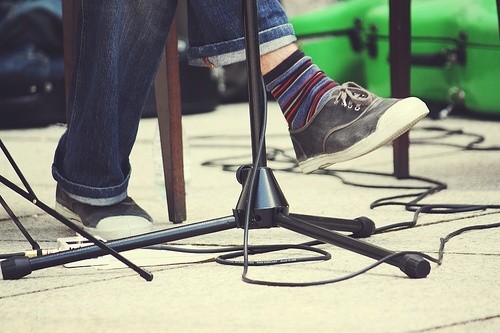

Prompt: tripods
xmin=1 ymin=1 xmax=433 ymax=281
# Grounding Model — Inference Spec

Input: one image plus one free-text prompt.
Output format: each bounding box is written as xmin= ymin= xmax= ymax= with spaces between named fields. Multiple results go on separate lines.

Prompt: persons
xmin=51 ymin=0 xmax=431 ymax=241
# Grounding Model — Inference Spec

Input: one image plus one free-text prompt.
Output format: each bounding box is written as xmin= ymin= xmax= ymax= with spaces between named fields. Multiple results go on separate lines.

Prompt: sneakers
xmin=288 ymin=82 xmax=429 ymax=175
xmin=54 ymin=182 xmax=155 ymax=240
